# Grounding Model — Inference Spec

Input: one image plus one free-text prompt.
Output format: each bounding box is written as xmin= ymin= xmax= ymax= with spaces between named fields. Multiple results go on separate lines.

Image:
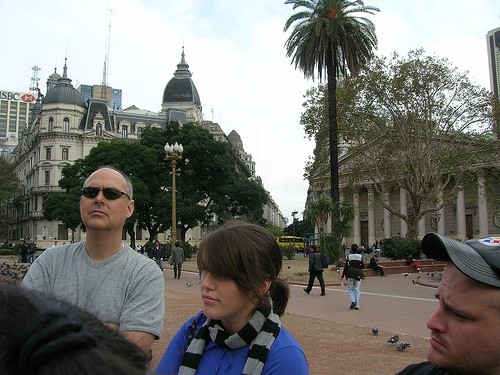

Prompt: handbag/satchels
xmin=320 ymin=255 xmax=328 ymax=268
xmin=346 ymin=267 xmax=363 ymax=281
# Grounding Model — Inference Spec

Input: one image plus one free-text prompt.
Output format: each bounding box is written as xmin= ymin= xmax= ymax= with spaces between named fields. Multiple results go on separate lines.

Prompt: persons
xmin=392 ymin=232 xmax=500 ymax=375
xmin=368 ymin=254 xmax=386 ymax=276
xmin=151 ymin=239 xmax=164 ymax=271
xmin=410 ymin=259 xmax=420 ymax=273
xmin=339 ymin=243 xmax=365 ymax=309
xmin=170 ymin=242 xmax=184 ymax=279
xmin=351 ymin=238 xmax=384 ymax=255
xmin=129 ymin=239 xmax=148 ymax=257
xmin=0 ymin=280 xmax=148 ymax=375
xmin=405 ymin=254 xmax=412 ymax=265
xmin=19 ymin=166 xmax=166 ymax=351
xmin=153 ymin=221 xmax=309 ymax=375
xmin=3 ymin=236 xmax=66 ymax=263
xmin=302 ymin=244 xmax=325 ymax=296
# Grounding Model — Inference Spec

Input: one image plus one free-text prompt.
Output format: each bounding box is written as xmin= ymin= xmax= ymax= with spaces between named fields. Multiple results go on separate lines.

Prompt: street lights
xmin=429 ymin=209 xmax=443 ymax=233
xmin=163 ymin=141 xmax=184 ymax=264
xmin=290 ymin=210 xmax=299 ymax=253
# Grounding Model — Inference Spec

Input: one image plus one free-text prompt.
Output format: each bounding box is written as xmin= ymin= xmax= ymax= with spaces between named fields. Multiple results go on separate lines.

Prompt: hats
xmin=420 ymin=232 xmax=500 ymax=287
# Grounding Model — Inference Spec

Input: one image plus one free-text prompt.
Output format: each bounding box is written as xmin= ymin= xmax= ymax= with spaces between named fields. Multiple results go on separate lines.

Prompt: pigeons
xmin=395 ymin=342 xmax=412 ymax=352
xmin=426 ymin=272 xmax=435 ymax=277
xmin=185 ymin=282 xmax=194 ymax=288
xmin=412 ymin=276 xmax=421 ymax=285
xmin=404 ymin=274 xmax=408 ymax=277
xmin=371 ymin=326 xmax=379 ymax=336
xmin=386 ymin=334 xmax=400 ymax=346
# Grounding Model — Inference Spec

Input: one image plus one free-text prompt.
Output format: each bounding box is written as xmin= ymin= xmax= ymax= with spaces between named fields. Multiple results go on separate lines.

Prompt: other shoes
xmin=355 ymin=307 xmax=360 ymax=310
xmin=303 ymin=288 xmax=309 ymax=294
xmin=320 ymin=293 xmax=325 ymax=295
xmin=350 ymin=302 xmax=356 ymax=308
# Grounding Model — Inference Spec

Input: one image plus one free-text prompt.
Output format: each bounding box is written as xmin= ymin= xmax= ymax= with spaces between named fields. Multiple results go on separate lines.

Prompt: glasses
xmin=80 ymin=186 xmax=131 ymax=201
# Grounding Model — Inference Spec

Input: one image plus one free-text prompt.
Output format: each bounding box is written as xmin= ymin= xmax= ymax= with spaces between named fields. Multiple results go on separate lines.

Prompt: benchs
xmin=362 ymin=259 xmax=448 ymax=276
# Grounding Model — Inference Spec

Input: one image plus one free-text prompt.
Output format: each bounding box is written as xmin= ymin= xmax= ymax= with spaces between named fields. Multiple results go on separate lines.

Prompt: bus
xmin=274 ymin=234 xmax=308 ymax=253
